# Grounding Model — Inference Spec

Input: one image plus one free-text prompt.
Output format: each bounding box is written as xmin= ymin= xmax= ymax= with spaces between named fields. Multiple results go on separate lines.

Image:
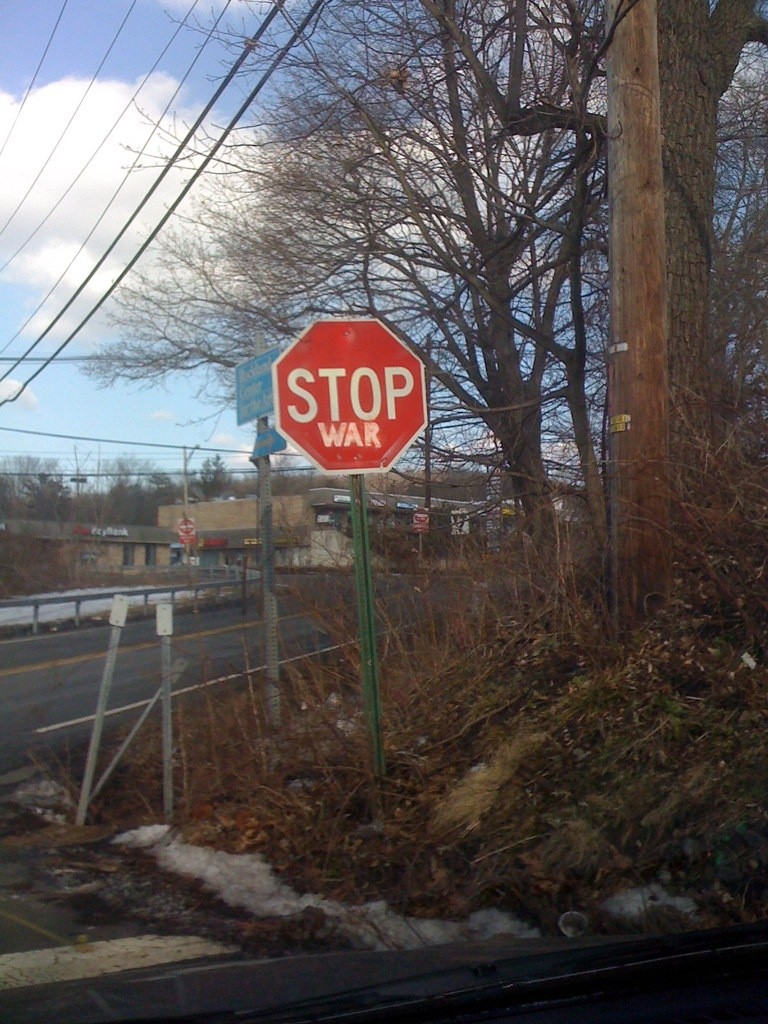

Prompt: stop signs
xmin=179 ymin=519 xmax=195 ymax=535
xmin=414 ymin=508 xmax=428 ymax=523
xmin=271 ymin=319 xmax=429 ymax=474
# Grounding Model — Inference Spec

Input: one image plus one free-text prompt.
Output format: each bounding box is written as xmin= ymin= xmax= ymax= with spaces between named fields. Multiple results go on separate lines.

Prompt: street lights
xmin=181 ymin=445 xmax=201 ymax=576
